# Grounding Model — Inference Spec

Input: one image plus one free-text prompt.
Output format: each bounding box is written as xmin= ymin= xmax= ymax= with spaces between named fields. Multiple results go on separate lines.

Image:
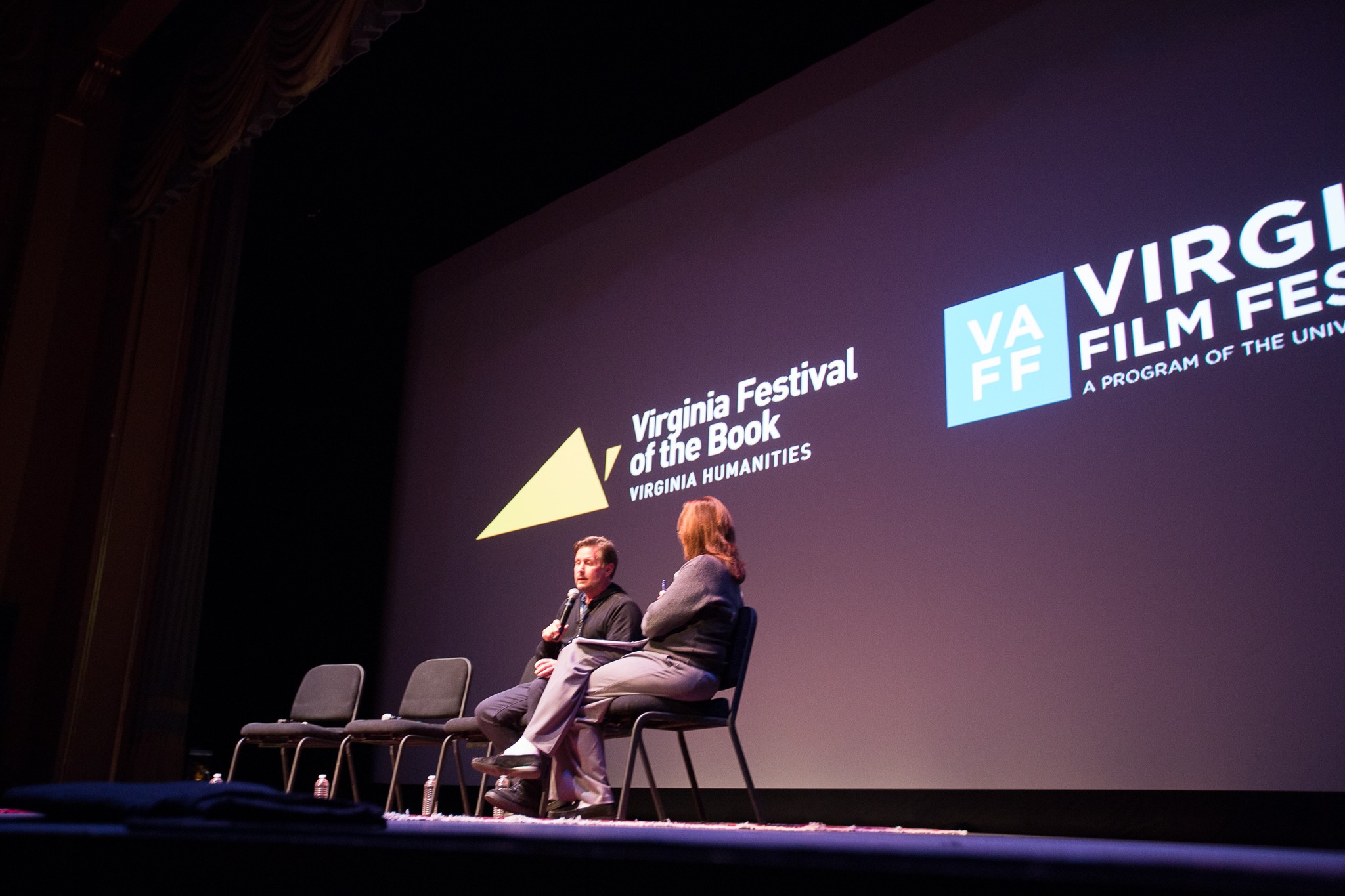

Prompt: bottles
xmin=422 ymin=775 xmax=438 ymax=815
xmin=313 ymin=774 xmax=329 ymax=800
xmin=493 ymin=775 xmax=510 ymax=817
xmin=209 ymin=773 xmax=223 ymax=784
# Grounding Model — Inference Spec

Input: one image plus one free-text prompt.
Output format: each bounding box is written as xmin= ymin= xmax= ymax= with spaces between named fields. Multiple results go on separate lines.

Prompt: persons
xmin=471 ymin=495 xmax=746 ymax=820
xmin=474 ymin=536 xmax=643 ymax=817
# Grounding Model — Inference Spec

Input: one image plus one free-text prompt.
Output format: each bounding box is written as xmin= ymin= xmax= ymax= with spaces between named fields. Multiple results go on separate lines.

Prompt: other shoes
xmin=484 ymin=777 xmax=543 ymax=818
xmin=472 ymin=754 xmax=542 ymax=779
xmin=547 ymin=803 xmax=615 ymax=820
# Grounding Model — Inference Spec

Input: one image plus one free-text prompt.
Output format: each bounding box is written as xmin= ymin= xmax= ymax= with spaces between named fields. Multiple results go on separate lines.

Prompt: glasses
xmin=675 ymin=530 xmax=682 ymax=537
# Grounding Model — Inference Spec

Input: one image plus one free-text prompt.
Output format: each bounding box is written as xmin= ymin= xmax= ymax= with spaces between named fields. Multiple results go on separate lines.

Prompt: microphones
xmin=552 ymin=588 xmax=580 ymax=640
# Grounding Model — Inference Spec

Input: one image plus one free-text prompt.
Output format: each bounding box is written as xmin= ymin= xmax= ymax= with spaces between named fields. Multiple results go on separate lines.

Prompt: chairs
xmin=329 ymin=655 xmax=472 ymax=817
xmin=428 ymin=656 xmax=536 ymax=820
xmin=225 ymin=662 xmax=365 ymax=803
xmin=607 ymin=605 xmax=762 ymax=825
